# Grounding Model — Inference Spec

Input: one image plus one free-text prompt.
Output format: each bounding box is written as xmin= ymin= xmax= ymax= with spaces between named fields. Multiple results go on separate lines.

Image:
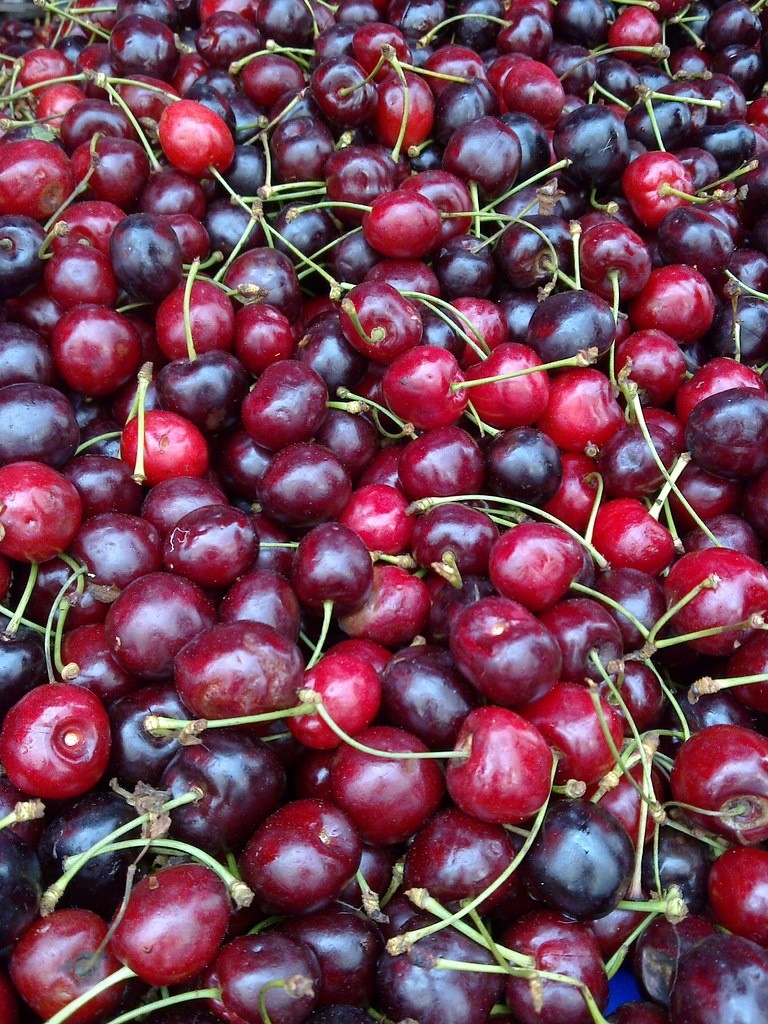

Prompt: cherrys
xmin=0 ymin=1 xmax=768 ymax=1024
